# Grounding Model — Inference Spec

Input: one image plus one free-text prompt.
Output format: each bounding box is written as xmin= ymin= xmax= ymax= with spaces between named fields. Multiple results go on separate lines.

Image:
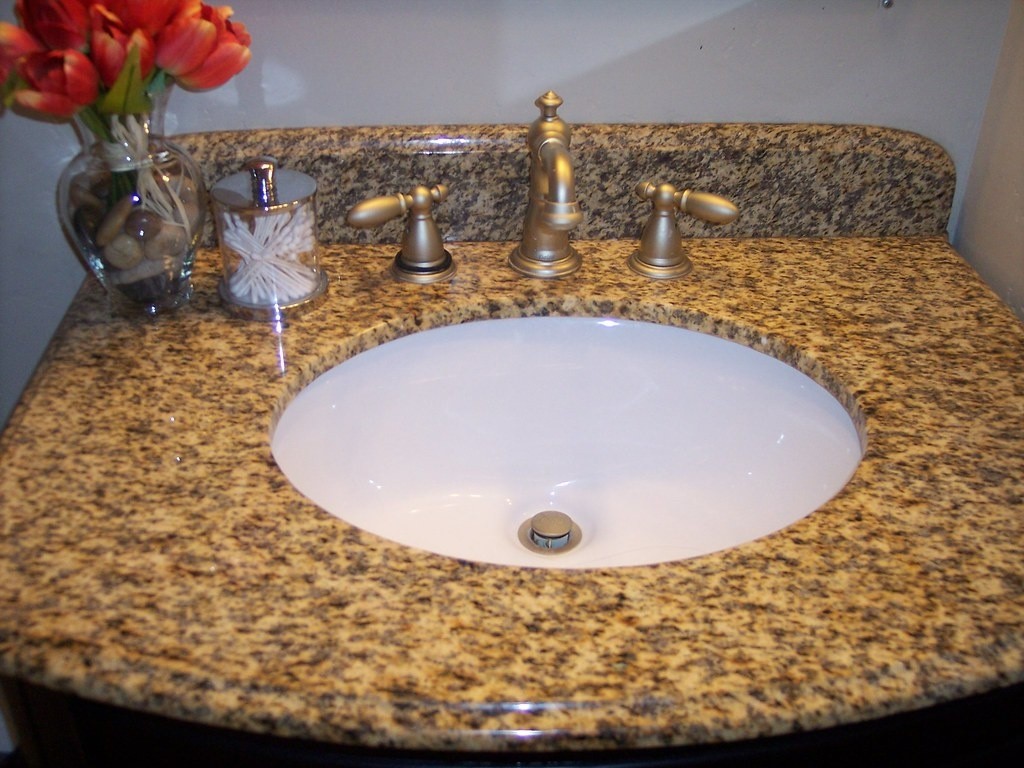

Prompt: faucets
xmin=508 ymin=91 xmax=583 ymax=277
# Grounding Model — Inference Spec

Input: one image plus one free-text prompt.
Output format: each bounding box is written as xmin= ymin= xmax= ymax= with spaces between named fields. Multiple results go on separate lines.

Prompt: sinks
xmin=271 ymin=299 xmax=868 ymax=568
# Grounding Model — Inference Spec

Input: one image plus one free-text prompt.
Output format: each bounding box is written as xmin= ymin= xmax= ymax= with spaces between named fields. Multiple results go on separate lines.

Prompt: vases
xmin=57 ymin=131 xmax=207 ymax=317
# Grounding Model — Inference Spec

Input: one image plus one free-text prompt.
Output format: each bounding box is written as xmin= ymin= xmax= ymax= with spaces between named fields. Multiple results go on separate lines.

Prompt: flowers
xmin=0 ymin=1 xmax=252 ymax=116
